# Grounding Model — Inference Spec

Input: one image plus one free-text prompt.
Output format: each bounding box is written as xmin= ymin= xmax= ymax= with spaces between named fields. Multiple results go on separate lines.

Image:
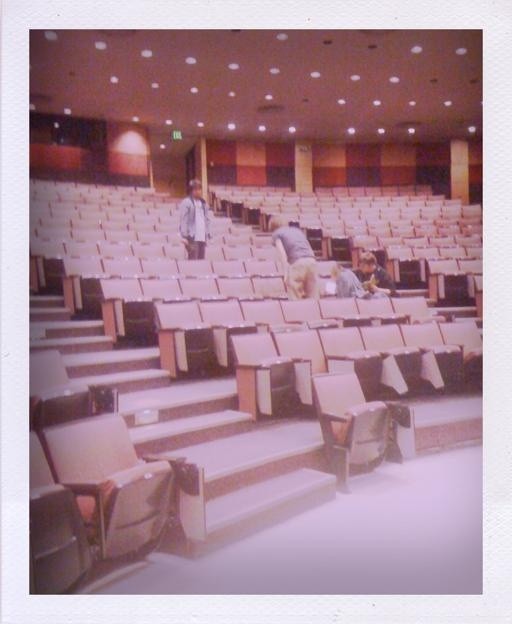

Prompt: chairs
xmin=32 ymin=326 xmax=178 ymax=595
xmin=32 ymin=178 xmax=483 ymax=492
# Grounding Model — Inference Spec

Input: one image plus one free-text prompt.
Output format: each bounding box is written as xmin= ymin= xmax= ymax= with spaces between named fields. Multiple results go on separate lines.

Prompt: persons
xmin=271 ymin=221 xmax=319 ymax=300
xmin=179 ymin=178 xmax=212 ymax=260
xmin=352 ymin=251 xmax=396 ymax=299
